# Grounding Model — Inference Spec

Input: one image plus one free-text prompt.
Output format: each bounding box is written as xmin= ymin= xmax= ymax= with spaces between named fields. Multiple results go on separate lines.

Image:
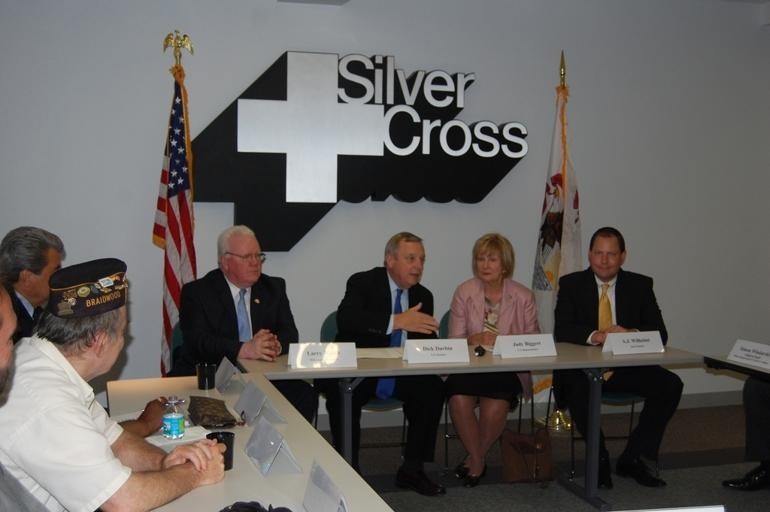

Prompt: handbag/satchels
xmin=499 ymin=429 xmax=556 ymax=489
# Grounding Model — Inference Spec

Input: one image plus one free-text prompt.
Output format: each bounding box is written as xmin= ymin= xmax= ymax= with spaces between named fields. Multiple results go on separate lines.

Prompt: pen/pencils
xmin=142 ymin=400 xmax=185 ymax=411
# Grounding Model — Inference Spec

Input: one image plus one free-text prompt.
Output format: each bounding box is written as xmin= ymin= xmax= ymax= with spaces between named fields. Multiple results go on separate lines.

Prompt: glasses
xmin=223 ymin=250 xmax=266 ymax=264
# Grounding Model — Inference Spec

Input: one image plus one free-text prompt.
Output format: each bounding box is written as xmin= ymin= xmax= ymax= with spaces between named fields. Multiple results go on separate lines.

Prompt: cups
xmin=196 ymin=361 xmax=216 ymax=390
xmin=206 ymin=431 xmax=235 ymax=471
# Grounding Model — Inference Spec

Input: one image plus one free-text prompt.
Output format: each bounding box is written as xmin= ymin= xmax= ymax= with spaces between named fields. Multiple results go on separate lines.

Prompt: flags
xmin=150 ymin=64 xmax=197 ymax=375
xmin=528 ymin=86 xmax=583 ymax=395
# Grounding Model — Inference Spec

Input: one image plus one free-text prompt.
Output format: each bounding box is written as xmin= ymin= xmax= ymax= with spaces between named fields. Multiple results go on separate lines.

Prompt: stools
xmin=540 ymin=383 xmax=648 ymax=477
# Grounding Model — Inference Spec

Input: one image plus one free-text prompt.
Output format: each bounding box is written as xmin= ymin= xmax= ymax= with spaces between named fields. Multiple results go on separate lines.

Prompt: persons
xmin=313 ymin=231 xmax=446 ymax=497
xmin=443 ymin=232 xmax=540 ymax=489
xmin=551 ymin=228 xmax=684 ymax=489
xmin=0 ymin=225 xmax=66 ymax=334
xmin=721 ymin=374 xmax=770 ymax=492
xmin=0 ymin=258 xmax=228 ymax=512
xmin=164 ymin=224 xmax=314 ymax=423
xmin=0 ymin=284 xmax=20 ymax=408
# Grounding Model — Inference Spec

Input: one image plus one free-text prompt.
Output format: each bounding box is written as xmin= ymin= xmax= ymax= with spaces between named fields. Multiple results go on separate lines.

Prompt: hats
xmin=48 ymin=258 xmax=130 ymax=318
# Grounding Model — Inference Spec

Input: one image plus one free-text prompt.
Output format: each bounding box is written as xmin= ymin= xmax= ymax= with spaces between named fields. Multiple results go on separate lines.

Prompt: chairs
xmin=314 ymin=309 xmax=410 ymax=462
xmin=435 ymin=304 xmax=530 ymax=471
xmin=164 ymin=318 xmax=192 ymax=369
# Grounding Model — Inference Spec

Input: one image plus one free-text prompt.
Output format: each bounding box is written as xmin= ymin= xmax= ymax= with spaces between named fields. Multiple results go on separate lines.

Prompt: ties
xmin=598 ymin=283 xmax=615 ymax=382
xmin=375 ymin=288 xmax=404 ymax=401
xmin=236 ymin=288 xmax=251 ymax=343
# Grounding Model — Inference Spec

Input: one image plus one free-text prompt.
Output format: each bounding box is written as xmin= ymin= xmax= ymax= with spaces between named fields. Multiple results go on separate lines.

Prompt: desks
xmin=104 ymin=368 xmax=395 ymax=512
xmin=705 ymin=341 xmax=770 ymax=387
xmin=237 ymin=330 xmax=709 ymax=512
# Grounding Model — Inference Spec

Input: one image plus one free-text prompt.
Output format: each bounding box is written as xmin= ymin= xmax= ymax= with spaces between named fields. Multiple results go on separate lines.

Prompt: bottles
xmin=162 ymin=394 xmax=186 ymax=441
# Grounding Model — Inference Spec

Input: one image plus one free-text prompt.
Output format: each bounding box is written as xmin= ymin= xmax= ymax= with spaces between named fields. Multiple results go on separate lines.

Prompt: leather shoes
xmin=594 ymin=448 xmax=613 ymax=489
xmin=395 ymin=463 xmax=447 ymax=497
xmin=464 ymin=464 xmax=488 ymax=488
xmin=721 ymin=463 xmax=770 ymax=492
xmin=453 ymin=460 xmax=468 ymax=481
xmin=616 ymin=456 xmax=668 ymax=487
